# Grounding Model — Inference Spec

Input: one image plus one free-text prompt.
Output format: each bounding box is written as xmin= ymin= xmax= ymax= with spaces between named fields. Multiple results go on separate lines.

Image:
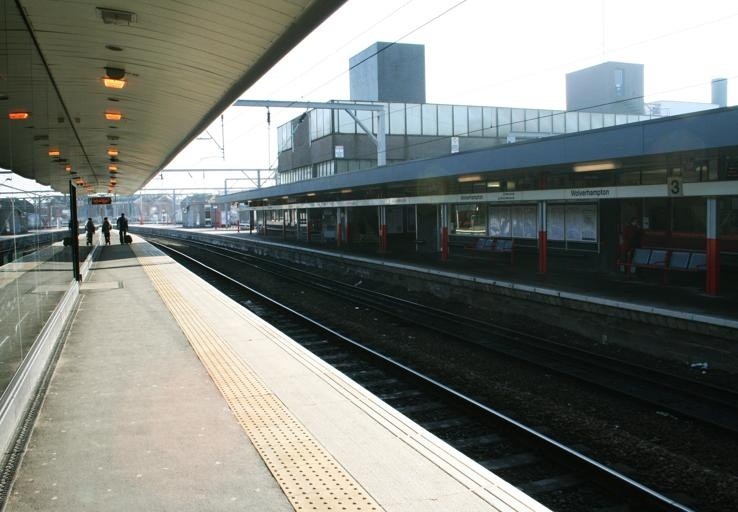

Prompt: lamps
xmin=103 ymin=66 xmax=127 ymax=174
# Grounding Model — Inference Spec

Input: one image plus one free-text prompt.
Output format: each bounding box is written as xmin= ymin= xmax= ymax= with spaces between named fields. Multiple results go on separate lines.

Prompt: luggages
xmin=124 ymin=231 xmax=132 ymax=244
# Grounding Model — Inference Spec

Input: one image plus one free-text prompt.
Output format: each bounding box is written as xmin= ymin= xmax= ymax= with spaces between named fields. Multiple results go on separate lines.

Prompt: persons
xmin=101 ymin=217 xmax=113 ymax=248
xmin=116 ymin=212 xmax=129 ymax=246
xmin=84 ymin=217 xmax=95 ymax=247
xmin=68 ymin=217 xmax=79 ymax=247
